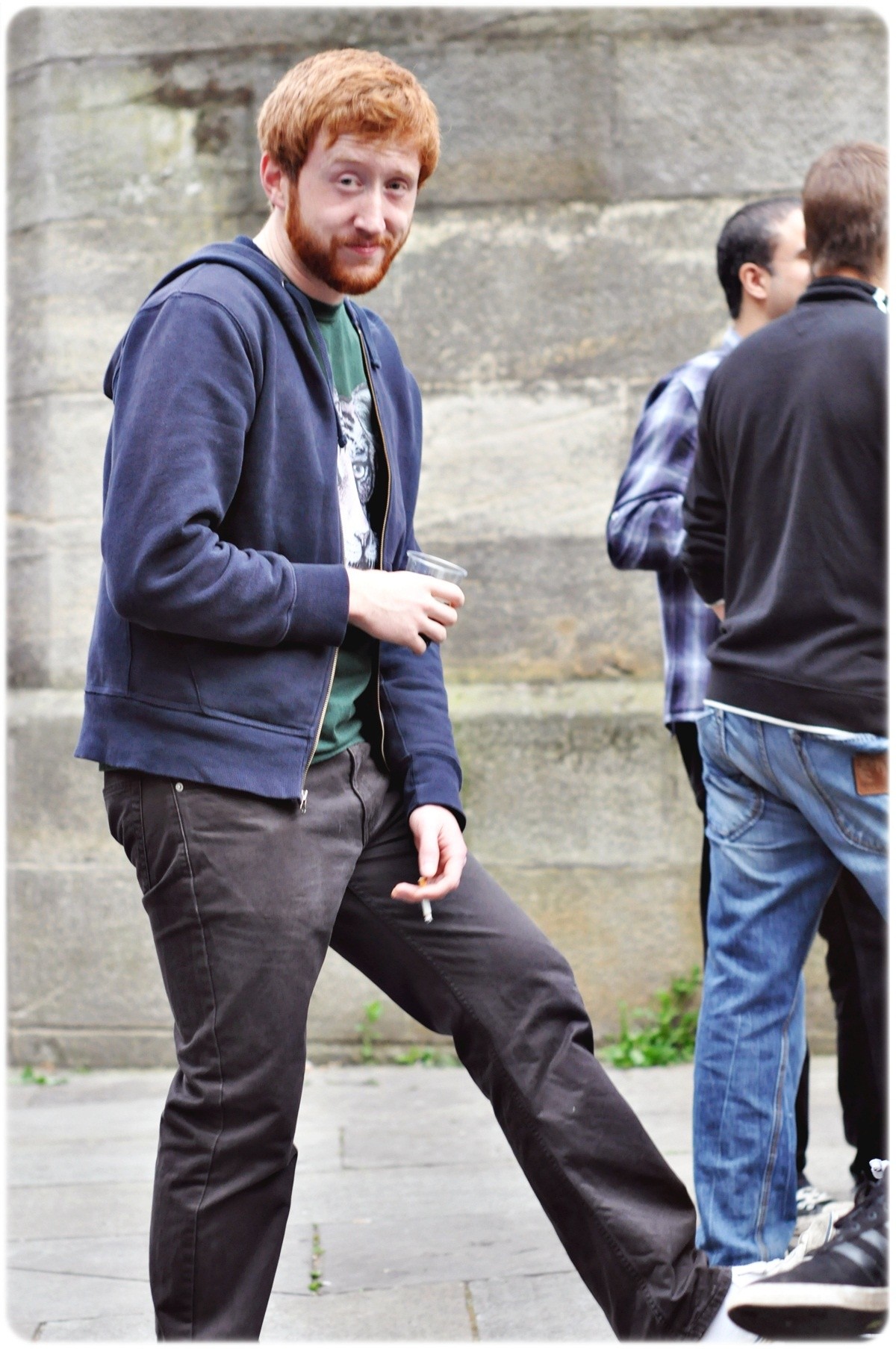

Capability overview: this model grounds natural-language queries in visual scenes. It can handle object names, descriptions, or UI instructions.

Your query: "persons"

[606,198,889,1210]
[732,1164,887,1336]
[681,139,888,1259]
[77,46,838,1344]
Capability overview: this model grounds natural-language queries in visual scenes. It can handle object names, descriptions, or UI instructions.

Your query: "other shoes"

[795,1181,844,1235]
[705,1212,836,1345]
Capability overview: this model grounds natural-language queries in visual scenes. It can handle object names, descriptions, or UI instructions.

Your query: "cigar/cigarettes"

[417,876,432,924]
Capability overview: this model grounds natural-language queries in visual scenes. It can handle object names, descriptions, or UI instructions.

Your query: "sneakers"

[725,1162,888,1339]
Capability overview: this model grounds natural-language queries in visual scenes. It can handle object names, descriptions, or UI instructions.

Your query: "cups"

[400,549,467,649]
